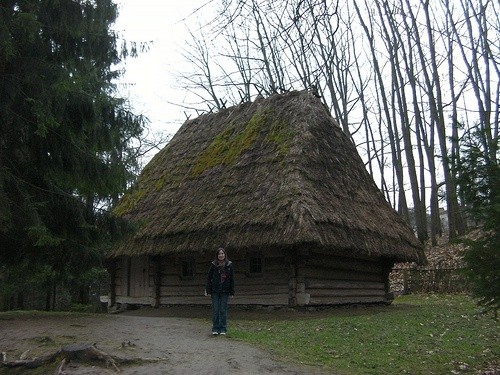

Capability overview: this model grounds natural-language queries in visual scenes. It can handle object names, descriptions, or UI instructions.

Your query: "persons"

[202,247,237,337]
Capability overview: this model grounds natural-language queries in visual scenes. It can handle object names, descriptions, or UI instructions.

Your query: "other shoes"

[212,332,225,335]
